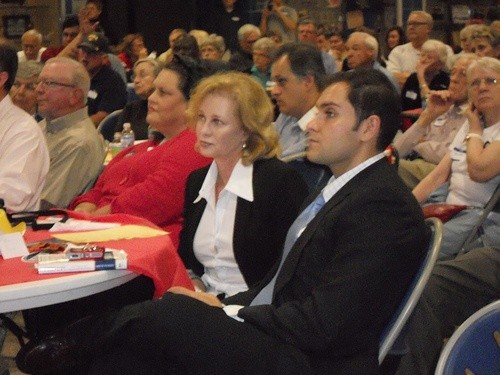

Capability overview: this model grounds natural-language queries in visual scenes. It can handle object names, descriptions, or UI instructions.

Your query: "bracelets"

[463,133,482,141]
[419,83,428,90]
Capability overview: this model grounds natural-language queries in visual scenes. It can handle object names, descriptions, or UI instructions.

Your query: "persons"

[27,68,215,328]
[0,0,397,214]
[72,71,307,320]
[17,67,432,375]
[388,0,500,375]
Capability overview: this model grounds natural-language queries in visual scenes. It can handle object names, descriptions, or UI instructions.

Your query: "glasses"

[33,79,75,90]
[469,79,497,88]
[407,22,427,26]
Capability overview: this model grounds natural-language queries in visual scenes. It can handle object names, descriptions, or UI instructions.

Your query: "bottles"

[111,132,124,158]
[121,122,135,149]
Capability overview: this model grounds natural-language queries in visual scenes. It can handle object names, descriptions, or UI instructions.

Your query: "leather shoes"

[15,310,113,375]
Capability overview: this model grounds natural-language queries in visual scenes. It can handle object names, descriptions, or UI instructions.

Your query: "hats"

[78,32,110,52]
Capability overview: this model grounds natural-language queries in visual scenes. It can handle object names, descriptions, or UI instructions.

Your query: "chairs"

[434,301,500,375]
[378,217,444,364]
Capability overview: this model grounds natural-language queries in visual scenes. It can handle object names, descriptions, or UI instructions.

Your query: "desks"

[0,207,195,348]
